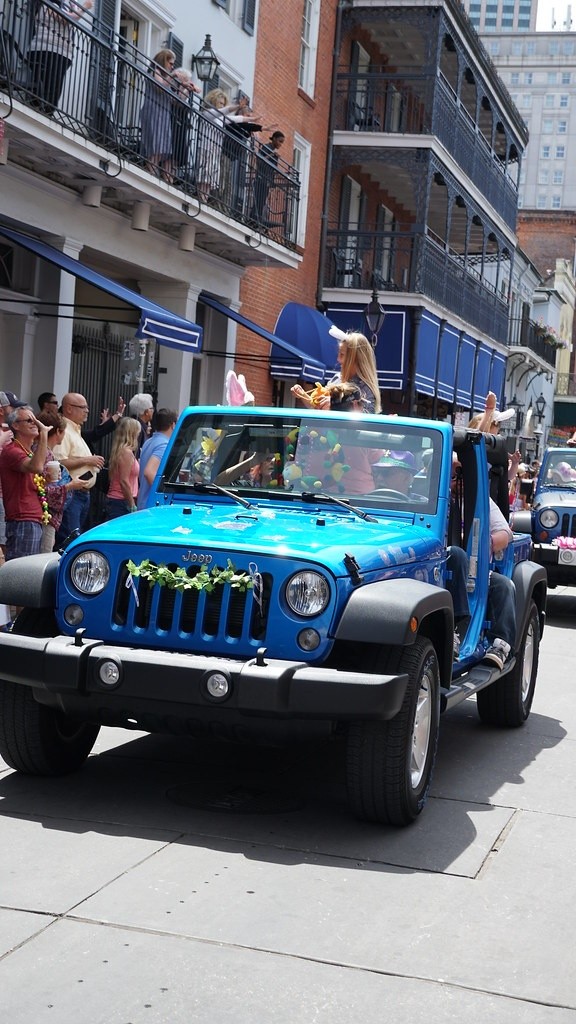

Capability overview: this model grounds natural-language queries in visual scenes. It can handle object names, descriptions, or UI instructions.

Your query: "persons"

[314,382,376,497]
[547,462,576,483]
[292,327,381,414]
[213,447,275,489]
[467,391,520,481]
[0,392,177,625]
[451,452,517,670]
[140,50,201,182]
[371,450,471,661]
[196,90,284,225]
[28,0,93,115]
[186,371,254,482]
[509,461,542,511]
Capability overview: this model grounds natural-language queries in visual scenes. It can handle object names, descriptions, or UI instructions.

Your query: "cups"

[93,466,100,473]
[46,461,61,482]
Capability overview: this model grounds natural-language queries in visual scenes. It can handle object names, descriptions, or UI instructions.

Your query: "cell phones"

[79,470,93,481]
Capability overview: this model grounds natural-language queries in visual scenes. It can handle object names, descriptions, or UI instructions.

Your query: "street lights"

[533,392,548,459]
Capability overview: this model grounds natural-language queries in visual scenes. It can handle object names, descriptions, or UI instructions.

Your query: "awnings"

[269,303,344,381]
[0,229,203,353]
[199,296,326,383]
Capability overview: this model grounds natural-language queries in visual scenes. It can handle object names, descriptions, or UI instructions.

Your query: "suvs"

[1,403,548,831]
[531,446,576,589]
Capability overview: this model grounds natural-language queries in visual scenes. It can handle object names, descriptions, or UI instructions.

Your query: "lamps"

[191,34,220,81]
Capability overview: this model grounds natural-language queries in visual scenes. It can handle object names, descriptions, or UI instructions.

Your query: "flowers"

[126,558,252,592]
[551,536,576,549]
[535,317,566,350]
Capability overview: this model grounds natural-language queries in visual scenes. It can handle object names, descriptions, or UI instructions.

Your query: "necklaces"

[15,439,52,525]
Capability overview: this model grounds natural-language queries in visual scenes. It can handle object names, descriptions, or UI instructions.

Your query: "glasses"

[450,475,456,481]
[492,421,500,426]
[149,407,155,412]
[70,404,89,409]
[15,418,33,423]
[46,401,58,405]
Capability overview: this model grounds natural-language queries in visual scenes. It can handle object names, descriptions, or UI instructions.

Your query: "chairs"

[355,103,381,131]
[97,108,220,204]
[411,476,427,496]
[243,178,286,229]
[0,29,47,110]
[332,248,399,292]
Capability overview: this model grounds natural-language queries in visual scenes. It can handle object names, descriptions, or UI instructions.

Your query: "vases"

[545,338,551,344]
[537,327,543,336]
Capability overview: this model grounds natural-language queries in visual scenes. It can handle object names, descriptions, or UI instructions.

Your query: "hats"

[0,391,28,407]
[479,408,515,422]
[567,431,576,444]
[330,385,372,404]
[370,449,419,476]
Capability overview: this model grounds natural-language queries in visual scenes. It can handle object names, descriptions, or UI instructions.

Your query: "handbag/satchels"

[94,453,111,494]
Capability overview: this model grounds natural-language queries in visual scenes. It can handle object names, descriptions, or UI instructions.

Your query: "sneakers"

[453,627,460,657]
[482,638,511,670]
